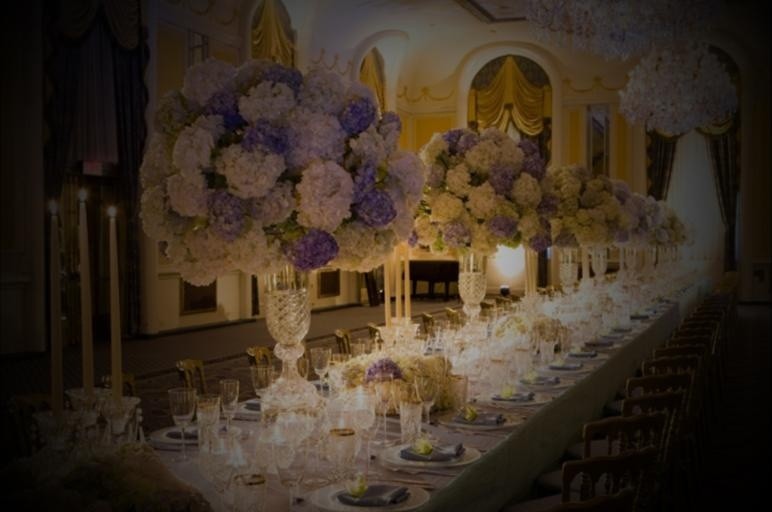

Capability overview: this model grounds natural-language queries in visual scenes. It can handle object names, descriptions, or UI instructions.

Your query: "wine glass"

[167,387,196,466]
[196,393,222,460]
[218,255,691,512]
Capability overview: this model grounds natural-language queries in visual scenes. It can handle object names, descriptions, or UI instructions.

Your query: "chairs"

[509,281,736,509]
[128,277,612,439]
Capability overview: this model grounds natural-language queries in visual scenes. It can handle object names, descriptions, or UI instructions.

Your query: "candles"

[107,207,123,417]
[47,200,63,417]
[76,189,94,408]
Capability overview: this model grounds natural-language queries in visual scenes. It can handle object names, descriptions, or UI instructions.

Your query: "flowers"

[550,164,684,251]
[405,124,552,256]
[617,59,737,138]
[138,59,426,274]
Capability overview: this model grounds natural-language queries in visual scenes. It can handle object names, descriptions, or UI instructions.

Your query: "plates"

[150,423,243,445]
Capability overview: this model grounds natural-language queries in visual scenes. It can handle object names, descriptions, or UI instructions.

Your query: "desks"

[121,273,715,512]
